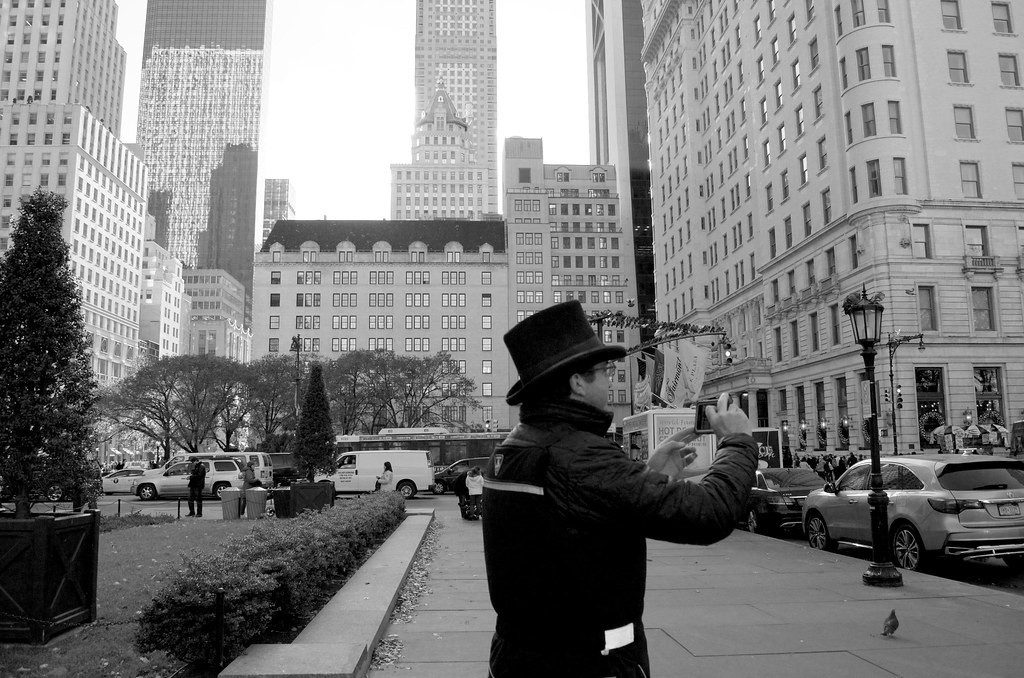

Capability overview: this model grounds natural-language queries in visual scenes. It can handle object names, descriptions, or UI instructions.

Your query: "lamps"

[885,408,893,428]
[783,414,853,434]
[962,406,972,426]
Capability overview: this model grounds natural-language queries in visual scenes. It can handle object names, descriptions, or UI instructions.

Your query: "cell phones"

[694,400,729,433]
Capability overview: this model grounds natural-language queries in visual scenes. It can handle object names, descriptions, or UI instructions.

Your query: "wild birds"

[882,609,900,637]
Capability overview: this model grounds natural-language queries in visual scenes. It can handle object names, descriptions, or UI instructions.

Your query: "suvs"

[801,453,1024,573]
[269,453,302,487]
[0,454,103,502]
[428,457,489,495]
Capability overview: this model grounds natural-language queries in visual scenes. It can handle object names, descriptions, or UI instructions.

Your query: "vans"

[141,452,274,496]
[313,450,435,500]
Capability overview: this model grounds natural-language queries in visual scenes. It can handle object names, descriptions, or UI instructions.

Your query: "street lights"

[843,281,904,588]
[289,333,303,437]
[885,330,925,454]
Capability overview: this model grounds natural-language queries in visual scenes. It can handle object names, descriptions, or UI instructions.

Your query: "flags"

[636,340,708,409]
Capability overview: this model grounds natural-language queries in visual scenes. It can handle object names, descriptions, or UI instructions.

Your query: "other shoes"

[476,516,479,520]
[196,514,202,517]
[185,511,195,517]
[469,515,473,521]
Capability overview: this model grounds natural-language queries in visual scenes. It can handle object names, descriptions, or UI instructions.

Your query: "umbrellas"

[931,423,1008,448]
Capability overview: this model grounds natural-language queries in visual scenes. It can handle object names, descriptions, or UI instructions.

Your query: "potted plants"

[289,363,338,518]
[0,184,102,647]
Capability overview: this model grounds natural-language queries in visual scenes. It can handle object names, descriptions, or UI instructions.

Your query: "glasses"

[592,360,616,379]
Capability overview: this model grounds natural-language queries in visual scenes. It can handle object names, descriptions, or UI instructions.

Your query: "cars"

[130,459,245,501]
[102,468,146,495]
[742,467,827,538]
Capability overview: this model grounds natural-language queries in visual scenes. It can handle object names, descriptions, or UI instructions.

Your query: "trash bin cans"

[273,490,290,518]
[220,487,241,519]
[245,487,268,519]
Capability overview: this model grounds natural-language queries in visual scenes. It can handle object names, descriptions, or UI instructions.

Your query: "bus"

[620,408,717,485]
[330,427,511,473]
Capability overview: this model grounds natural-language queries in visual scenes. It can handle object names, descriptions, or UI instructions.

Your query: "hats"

[246,461,255,467]
[502,300,627,406]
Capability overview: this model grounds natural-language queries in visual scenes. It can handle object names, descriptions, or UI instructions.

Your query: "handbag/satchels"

[829,462,833,470]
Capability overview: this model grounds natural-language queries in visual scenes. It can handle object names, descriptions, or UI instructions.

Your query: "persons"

[480,301,758,678]
[240,461,258,515]
[450,466,484,520]
[375,461,393,492]
[794,452,867,483]
[116,459,127,470]
[184,456,206,517]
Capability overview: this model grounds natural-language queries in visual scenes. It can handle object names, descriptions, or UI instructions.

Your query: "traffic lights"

[896,393,904,409]
[884,388,890,402]
